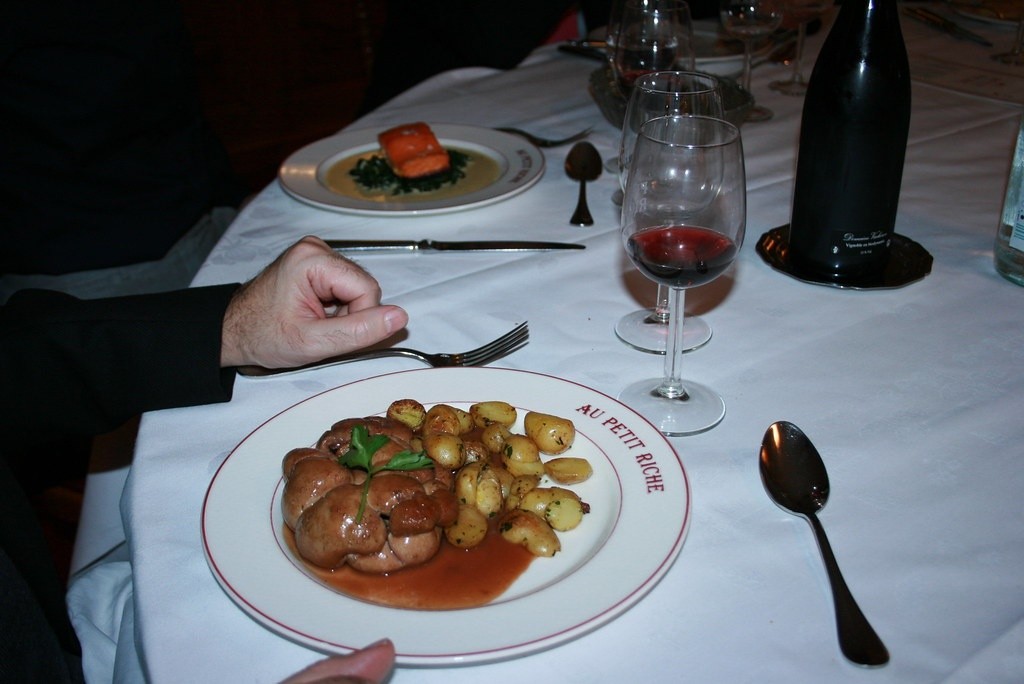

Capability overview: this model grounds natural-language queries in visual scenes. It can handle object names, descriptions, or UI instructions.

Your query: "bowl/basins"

[676,32,748,78]
[590,68,757,136]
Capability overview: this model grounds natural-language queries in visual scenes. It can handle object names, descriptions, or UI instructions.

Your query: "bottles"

[786,0,911,271]
[993,112,1024,288]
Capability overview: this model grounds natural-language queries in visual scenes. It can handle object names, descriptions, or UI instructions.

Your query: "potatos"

[386,399,592,556]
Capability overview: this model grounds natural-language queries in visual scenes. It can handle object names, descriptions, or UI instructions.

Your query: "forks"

[235,321,529,367]
[491,126,591,148]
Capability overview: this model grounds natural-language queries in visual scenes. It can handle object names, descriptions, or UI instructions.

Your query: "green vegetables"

[338,423,435,525]
[348,150,472,196]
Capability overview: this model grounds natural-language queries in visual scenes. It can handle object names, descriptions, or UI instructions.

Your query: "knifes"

[901,6,993,47]
[325,239,586,254]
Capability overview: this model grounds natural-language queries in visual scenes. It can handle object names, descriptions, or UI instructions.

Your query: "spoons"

[758,422,891,667]
[564,142,602,227]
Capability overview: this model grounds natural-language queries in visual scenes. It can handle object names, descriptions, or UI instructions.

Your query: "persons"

[0,233,408,684]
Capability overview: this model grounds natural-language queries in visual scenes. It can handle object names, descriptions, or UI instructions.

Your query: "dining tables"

[68,0,1023,684]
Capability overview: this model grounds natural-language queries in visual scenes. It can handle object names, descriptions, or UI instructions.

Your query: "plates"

[278,123,546,216]
[201,365,693,667]
[951,0,1024,26]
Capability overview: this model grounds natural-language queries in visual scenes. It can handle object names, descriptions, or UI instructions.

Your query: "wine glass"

[621,115,747,439]
[601,1,833,172]
[616,69,729,353]
